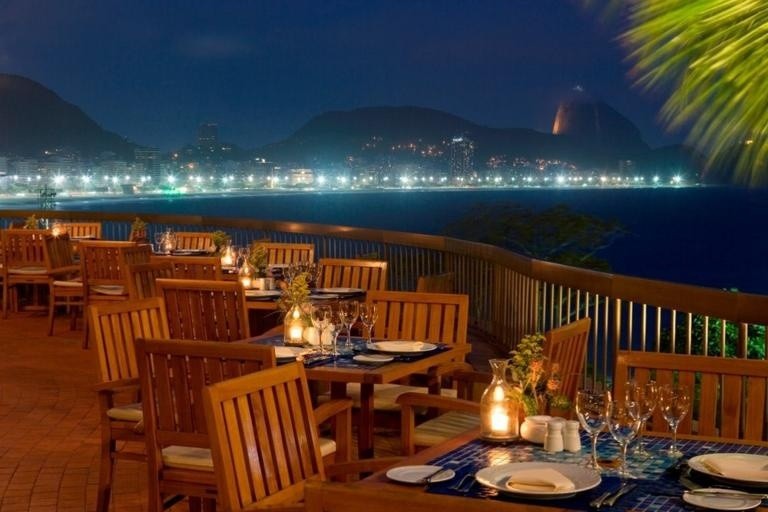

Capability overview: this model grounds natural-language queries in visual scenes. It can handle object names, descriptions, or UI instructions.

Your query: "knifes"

[589,481,637,509]
[684,490,768,501]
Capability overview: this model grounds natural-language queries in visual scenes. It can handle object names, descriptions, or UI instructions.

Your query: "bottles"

[519,415,582,453]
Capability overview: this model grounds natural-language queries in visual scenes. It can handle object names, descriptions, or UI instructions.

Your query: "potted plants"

[508,329,549,421]
[549,394,572,418]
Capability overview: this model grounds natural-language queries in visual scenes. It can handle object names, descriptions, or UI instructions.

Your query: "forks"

[678,478,700,490]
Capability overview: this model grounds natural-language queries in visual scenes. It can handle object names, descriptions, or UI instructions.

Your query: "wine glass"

[575,381,692,478]
[309,300,380,362]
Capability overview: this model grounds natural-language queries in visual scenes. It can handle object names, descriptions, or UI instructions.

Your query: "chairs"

[138,337,356,512]
[304,478,549,510]
[415,271,455,294]
[201,358,404,509]
[84,297,194,512]
[395,316,590,458]
[452,315,590,414]
[613,349,768,442]
[316,290,474,434]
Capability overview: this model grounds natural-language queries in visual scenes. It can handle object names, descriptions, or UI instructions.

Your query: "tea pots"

[479,358,523,444]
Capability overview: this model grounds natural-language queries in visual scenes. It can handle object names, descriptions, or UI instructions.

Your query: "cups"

[260,277,276,290]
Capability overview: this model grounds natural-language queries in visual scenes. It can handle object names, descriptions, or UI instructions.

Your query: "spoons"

[416,466,449,489]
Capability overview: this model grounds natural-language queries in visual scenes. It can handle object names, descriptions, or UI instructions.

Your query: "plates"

[366,340,438,355]
[687,452,768,483]
[383,464,456,484]
[682,488,762,511]
[474,462,603,501]
[352,354,394,364]
